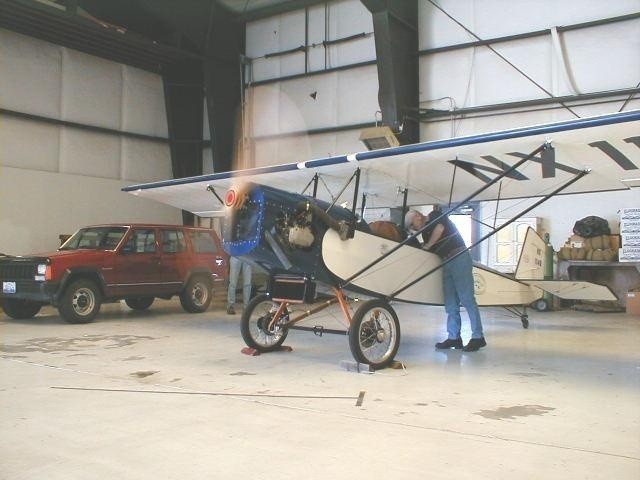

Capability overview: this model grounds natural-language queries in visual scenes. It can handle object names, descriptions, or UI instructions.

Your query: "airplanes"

[121,111,640,366]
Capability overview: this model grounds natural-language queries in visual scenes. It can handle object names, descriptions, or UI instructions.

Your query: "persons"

[403,209,487,353]
[225,253,252,315]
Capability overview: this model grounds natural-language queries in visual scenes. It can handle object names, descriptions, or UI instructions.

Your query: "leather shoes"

[227,309,235,314]
[463,337,486,352]
[435,338,463,349]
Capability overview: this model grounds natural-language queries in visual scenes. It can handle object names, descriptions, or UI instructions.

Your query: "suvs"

[0,223,230,323]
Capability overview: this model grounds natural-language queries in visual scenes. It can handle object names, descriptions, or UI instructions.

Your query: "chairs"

[122,231,164,252]
